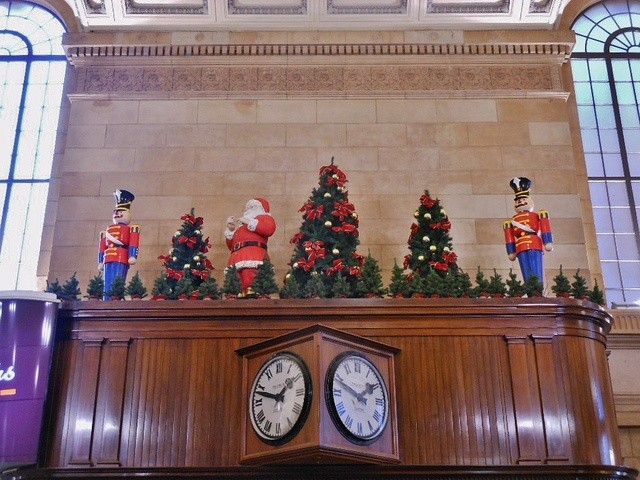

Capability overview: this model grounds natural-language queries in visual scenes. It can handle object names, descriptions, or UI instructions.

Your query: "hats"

[113,190,134,211]
[246,198,269,213]
[509,176,532,200]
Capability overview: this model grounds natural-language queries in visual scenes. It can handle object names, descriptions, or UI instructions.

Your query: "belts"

[108,244,128,248]
[516,232,537,238]
[230,241,268,254]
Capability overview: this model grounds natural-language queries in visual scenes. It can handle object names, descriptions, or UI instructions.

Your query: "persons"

[224,197,276,299]
[98,188,141,302]
[503,176,553,298]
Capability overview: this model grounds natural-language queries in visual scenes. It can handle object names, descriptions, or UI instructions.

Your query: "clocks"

[322,349,390,447]
[247,349,314,448]
